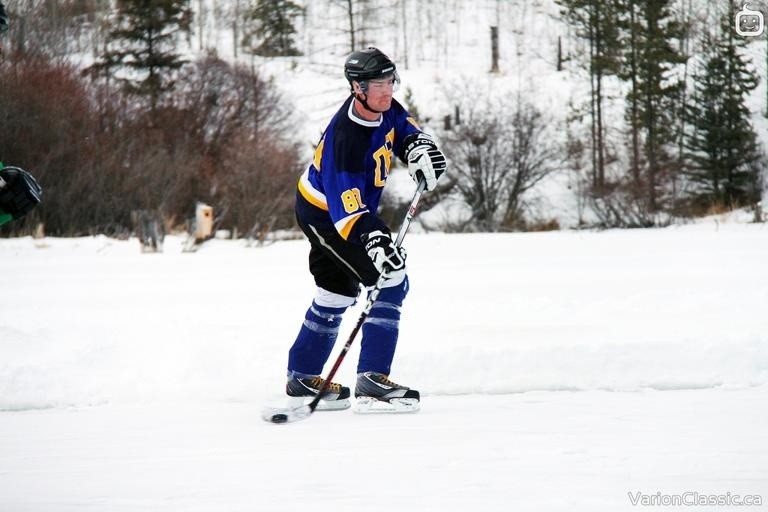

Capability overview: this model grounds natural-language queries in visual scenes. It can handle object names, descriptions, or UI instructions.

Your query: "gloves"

[397,132,448,194]
[358,229,407,279]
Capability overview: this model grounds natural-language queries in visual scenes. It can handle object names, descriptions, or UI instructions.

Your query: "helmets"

[344,47,396,82]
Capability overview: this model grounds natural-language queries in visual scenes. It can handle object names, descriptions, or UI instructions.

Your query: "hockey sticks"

[262,178,426,422]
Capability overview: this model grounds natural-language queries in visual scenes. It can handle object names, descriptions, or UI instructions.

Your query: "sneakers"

[286,369,350,402]
[355,371,419,400]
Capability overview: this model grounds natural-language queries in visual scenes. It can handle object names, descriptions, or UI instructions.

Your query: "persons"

[285,46,447,406]
[0,159,42,226]
